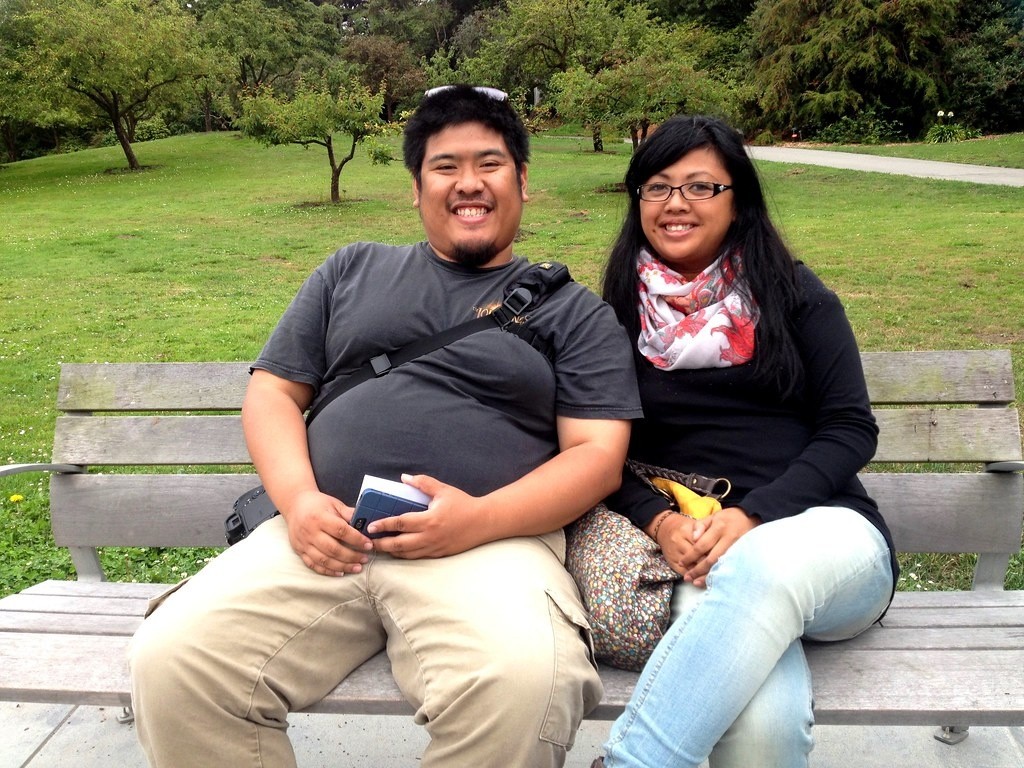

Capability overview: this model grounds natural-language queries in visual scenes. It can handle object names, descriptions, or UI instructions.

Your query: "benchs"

[0,349,1024,768]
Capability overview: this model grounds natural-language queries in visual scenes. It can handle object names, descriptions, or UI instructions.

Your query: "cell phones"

[348,489,428,539]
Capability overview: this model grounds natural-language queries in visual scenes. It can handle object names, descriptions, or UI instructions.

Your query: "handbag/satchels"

[224,484,281,547]
[566,457,732,674]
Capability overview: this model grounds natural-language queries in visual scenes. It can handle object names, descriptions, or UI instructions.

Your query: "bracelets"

[651,510,677,542]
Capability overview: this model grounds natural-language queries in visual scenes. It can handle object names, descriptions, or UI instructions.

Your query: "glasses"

[635,182,733,201]
[420,84,509,105]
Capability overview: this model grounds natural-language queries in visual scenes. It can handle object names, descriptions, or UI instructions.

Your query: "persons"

[591,114,901,765]
[122,84,650,768]
